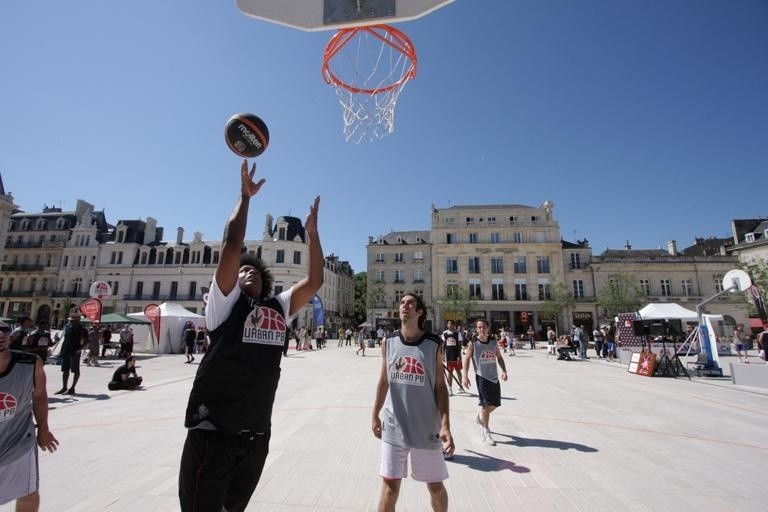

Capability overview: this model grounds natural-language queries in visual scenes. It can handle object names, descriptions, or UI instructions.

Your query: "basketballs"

[224,113,269,158]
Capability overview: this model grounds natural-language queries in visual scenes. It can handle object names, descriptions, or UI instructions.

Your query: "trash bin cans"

[744,336,752,350]
[367,339,375,348]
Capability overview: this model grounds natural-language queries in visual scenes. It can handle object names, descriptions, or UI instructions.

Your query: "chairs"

[570,344,577,360]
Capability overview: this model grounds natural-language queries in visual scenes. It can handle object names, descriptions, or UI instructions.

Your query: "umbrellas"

[68,312,151,325]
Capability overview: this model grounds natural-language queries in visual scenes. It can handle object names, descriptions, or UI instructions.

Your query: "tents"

[126,302,206,354]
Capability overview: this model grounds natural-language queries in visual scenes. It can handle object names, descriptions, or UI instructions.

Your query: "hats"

[66,313,81,321]
[600,324,607,329]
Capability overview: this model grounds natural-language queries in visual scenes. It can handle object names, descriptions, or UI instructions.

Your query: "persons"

[371,292,455,512]
[457,324,478,354]
[179,160,324,512]
[557,323,616,362]
[462,318,507,446]
[499,327,516,356]
[196,328,205,354]
[283,324,395,356]
[758,323,768,361]
[9,312,142,396]
[441,320,463,395]
[526,325,536,349]
[184,324,196,363]
[546,326,556,354]
[733,323,749,362]
[0,317,59,512]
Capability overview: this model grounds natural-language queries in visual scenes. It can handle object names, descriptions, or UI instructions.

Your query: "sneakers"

[448,387,453,395]
[55,387,75,396]
[738,357,749,363]
[457,384,463,394]
[480,429,497,446]
[185,357,195,363]
[596,354,617,362]
[474,413,490,432]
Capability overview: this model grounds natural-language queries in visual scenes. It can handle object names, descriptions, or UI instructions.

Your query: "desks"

[101,341,140,358]
[546,344,571,359]
